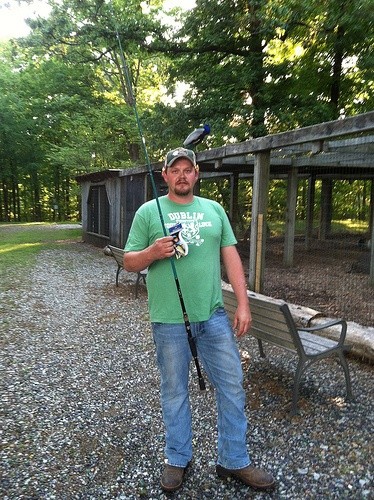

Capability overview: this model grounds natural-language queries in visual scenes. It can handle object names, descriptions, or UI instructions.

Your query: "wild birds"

[182,122,211,152]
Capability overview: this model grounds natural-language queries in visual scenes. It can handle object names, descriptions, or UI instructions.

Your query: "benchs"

[218,284,355,419]
[107,245,148,302]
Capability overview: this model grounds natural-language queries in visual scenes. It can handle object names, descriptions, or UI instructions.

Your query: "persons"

[124,147,274,491]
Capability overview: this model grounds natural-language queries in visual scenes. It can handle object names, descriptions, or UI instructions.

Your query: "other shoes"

[161,456,192,490]
[216,464,274,488]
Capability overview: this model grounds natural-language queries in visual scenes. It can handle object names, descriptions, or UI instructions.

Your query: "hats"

[165,147,197,170]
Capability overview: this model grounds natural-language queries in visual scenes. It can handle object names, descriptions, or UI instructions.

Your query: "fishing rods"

[115,33,207,392]
